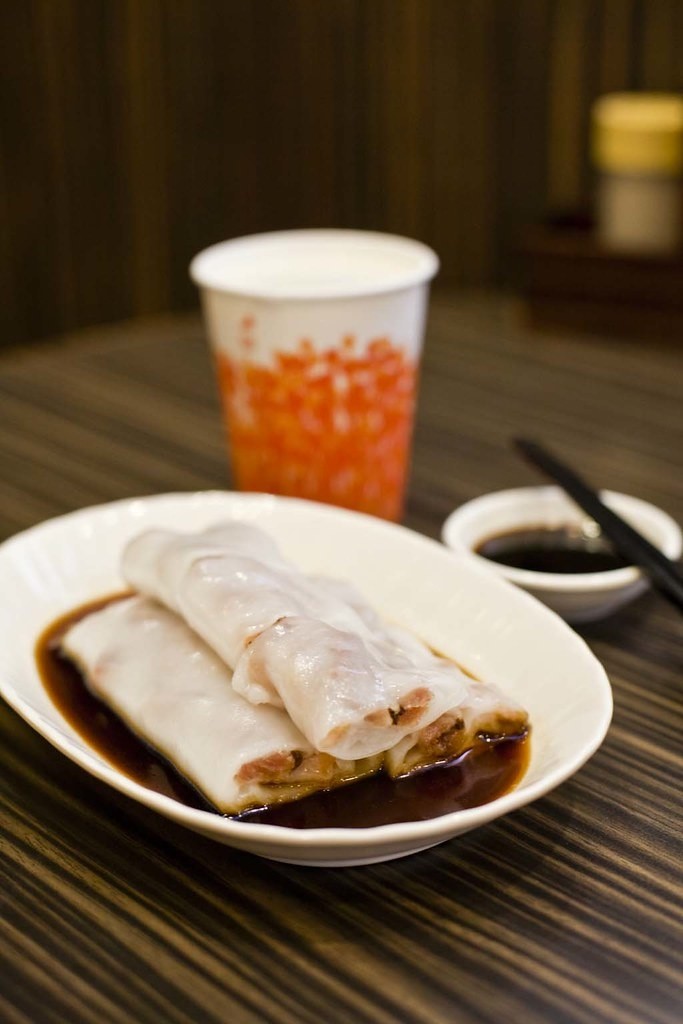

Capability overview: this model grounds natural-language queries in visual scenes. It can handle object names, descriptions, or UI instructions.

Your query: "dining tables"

[0,327,683,1024]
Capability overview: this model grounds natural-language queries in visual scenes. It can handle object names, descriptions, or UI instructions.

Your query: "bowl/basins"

[440,484,683,624]
[0,488,615,869]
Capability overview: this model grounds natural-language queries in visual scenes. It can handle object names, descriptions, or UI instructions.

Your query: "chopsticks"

[515,439,683,614]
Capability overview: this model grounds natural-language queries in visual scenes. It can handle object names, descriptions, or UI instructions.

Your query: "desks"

[494,220,683,350]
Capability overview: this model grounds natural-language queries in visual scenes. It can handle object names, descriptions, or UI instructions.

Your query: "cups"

[187,226,440,526]
